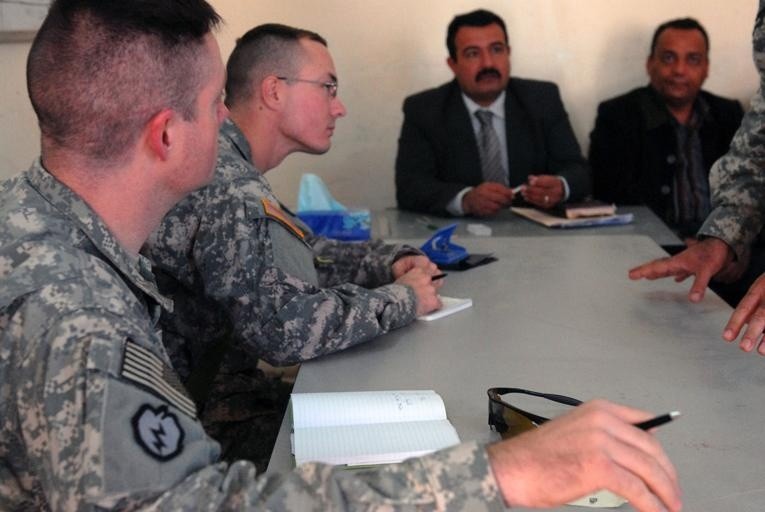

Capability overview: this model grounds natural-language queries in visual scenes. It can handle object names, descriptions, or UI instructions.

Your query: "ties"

[474,111,505,185]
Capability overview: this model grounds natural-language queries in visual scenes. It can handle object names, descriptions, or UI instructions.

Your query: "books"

[418,295,472,321]
[508,206,634,229]
[290,389,461,468]
[550,195,616,217]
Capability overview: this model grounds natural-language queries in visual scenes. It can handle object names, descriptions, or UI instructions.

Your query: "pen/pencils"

[636,410,683,431]
[511,184,526,194]
[431,273,448,281]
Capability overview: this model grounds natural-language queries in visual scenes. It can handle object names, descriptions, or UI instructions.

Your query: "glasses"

[487,385,584,440]
[277,76,337,97]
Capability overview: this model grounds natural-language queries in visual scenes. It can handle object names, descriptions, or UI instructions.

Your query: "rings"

[545,196,549,203]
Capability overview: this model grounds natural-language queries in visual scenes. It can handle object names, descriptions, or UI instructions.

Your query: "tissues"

[295,172,372,241]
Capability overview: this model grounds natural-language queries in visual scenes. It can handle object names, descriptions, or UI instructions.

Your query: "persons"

[0,0,681,512]
[139,24,445,474]
[588,17,765,310]
[396,9,592,219]
[629,0,765,356]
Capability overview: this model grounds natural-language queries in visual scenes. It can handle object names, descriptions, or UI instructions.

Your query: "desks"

[354,205,685,248]
[267,235,764,512]
